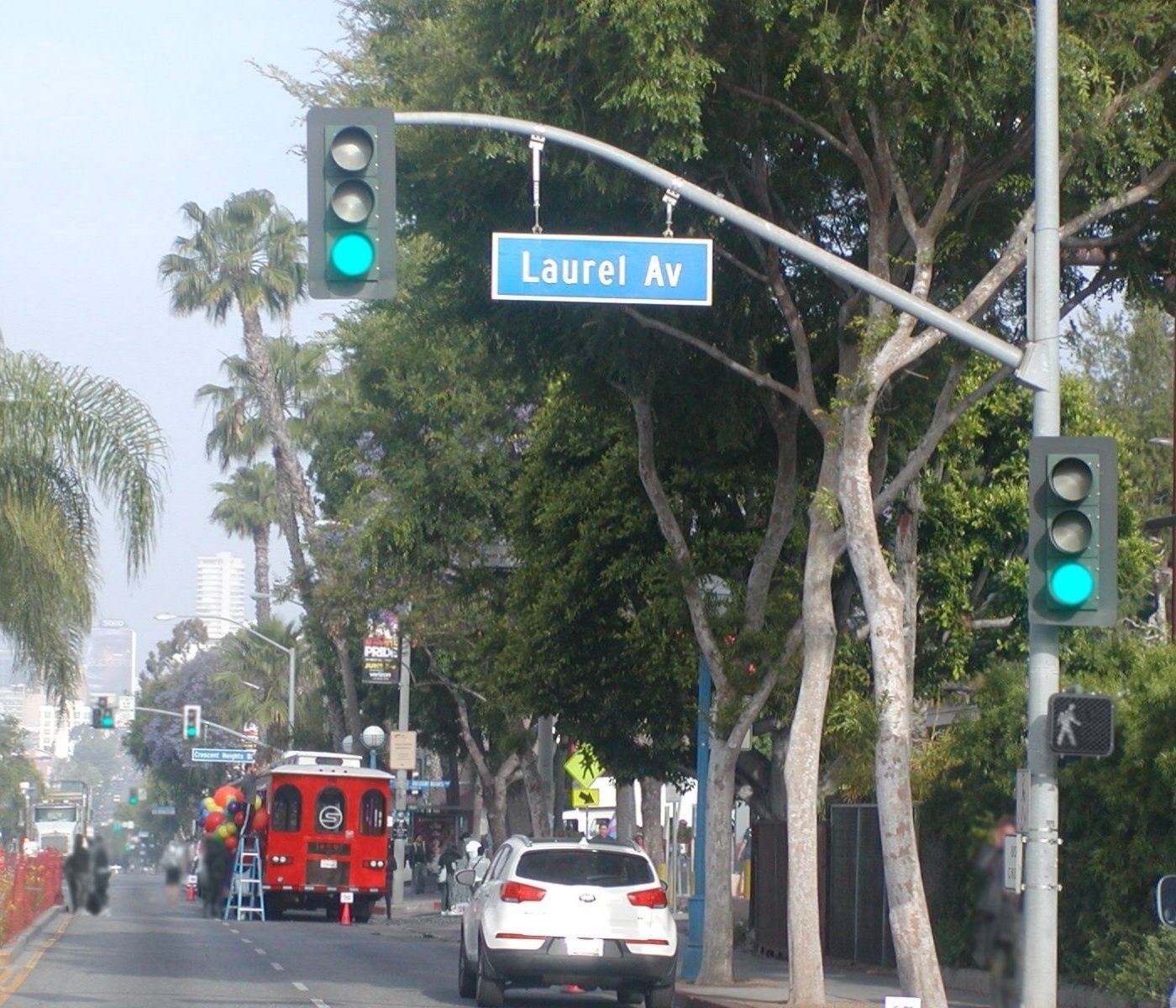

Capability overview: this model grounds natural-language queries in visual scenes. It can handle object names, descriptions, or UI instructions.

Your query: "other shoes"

[441,909,447,916]
[416,889,425,894]
[448,911,456,916]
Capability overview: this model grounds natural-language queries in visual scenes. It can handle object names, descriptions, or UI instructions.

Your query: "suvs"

[458,832,677,1007]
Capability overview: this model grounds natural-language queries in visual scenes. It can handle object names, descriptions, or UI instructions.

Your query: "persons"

[968,812,1076,1008]
[660,818,692,858]
[738,827,752,896]
[383,832,492,920]
[201,838,233,919]
[65,834,110,919]
[165,841,181,903]
[635,832,644,849]
[592,823,614,845]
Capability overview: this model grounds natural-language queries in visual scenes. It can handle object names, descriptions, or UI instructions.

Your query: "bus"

[223,750,396,923]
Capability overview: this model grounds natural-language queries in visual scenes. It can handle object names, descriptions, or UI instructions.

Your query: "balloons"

[198,787,268,867]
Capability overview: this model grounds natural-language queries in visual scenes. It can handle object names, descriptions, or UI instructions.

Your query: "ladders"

[225,834,264,921]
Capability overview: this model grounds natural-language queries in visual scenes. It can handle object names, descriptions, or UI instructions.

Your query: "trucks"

[12,779,96,861]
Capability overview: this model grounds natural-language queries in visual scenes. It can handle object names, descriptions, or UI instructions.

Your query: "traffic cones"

[186,885,196,902]
[340,903,351,926]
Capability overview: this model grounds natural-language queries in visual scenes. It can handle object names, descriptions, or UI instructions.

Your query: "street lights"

[359,725,390,769]
[153,613,297,750]
[314,519,412,919]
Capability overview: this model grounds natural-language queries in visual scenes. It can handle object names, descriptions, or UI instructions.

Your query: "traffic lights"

[1028,435,1118,627]
[183,704,201,740]
[92,697,113,729]
[307,106,397,299]
[129,787,138,805]
[1046,694,1115,757]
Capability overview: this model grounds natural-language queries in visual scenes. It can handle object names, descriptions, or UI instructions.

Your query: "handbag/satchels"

[435,865,447,884]
